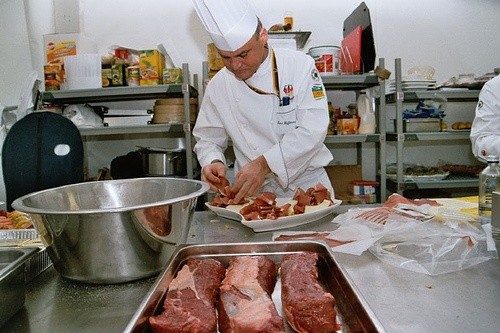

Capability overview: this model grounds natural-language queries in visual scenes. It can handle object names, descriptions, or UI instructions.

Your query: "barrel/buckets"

[309,45,343,76]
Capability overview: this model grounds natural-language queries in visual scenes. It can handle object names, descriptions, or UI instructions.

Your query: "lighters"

[283,97,290,106]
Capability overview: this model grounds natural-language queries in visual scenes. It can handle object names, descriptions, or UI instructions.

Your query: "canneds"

[127,66,139,87]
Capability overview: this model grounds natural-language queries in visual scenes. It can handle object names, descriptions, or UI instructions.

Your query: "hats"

[193,0,259,52]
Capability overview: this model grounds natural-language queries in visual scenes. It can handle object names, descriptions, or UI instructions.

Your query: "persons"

[192,0,336,205]
[470,72,500,164]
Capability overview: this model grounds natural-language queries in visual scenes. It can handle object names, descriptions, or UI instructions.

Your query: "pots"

[147,148,187,178]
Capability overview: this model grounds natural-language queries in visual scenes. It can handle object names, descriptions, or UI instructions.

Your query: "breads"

[451,122,471,128]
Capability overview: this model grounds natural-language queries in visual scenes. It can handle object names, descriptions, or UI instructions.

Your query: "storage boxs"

[158,52,166,85]
[42,31,99,89]
[138,49,160,85]
[390,119,442,133]
[347,184,373,196]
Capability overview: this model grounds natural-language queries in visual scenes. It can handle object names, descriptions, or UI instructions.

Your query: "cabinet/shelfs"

[375,57,482,196]
[202,57,387,204]
[30,62,200,180]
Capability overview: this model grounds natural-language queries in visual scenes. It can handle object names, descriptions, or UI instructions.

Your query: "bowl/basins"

[336,118,358,135]
[11,177,211,285]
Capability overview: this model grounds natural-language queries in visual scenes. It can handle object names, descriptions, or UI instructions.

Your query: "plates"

[203,198,342,232]
[377,169,449,182]
[442,164,487,176]
[389,77,437,92]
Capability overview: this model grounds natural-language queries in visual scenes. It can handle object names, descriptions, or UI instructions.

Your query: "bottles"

[356,91,376,134]
[479,155,500,224]
[326,101,335,134]
